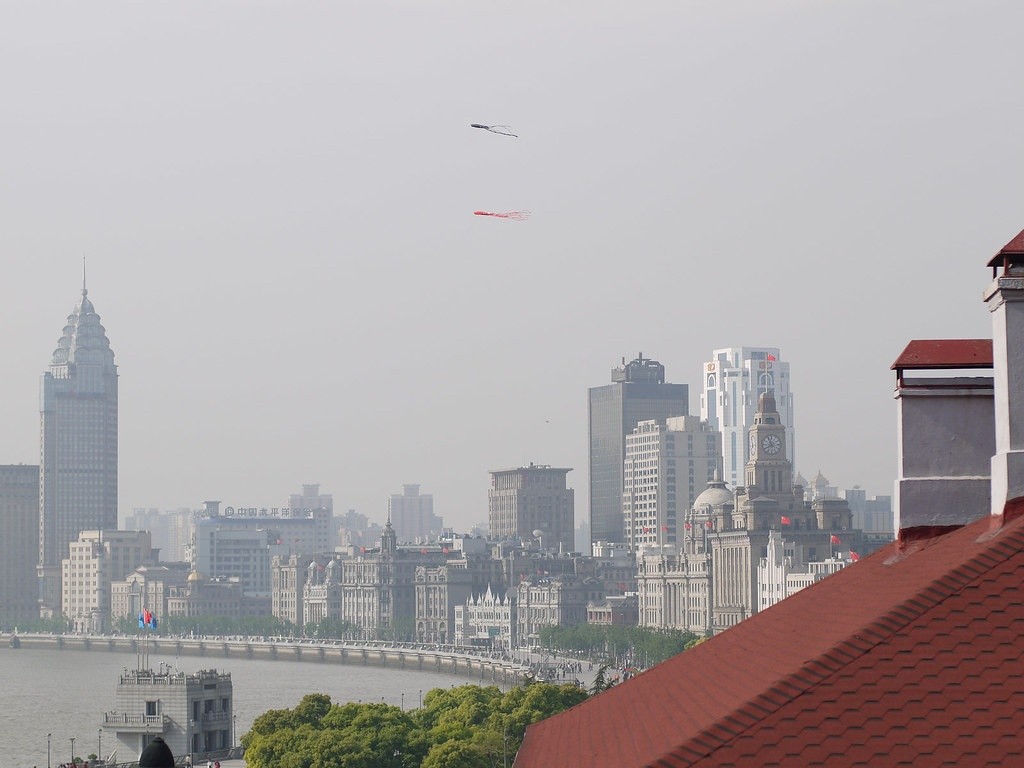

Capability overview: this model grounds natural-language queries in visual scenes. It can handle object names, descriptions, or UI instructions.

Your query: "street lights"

[47,732,51,768]
[419,690,422,711]
[402,693,405,711]
[97,729,103,765]
[145,722,151,747]
[233,714,238,747]
[190,717,195,768]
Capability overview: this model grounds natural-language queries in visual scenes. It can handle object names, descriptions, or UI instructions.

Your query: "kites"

[471,123,518,137]
[473,210,532,220]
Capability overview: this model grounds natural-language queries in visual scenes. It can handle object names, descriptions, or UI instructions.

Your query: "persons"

[208,758,212,768]
[553,653,643,689]
[186,755,191,768]
[215,762,220,768]
[59,761,89,768]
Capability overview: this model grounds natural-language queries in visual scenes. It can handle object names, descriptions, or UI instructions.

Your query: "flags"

[662,527,669,532]
[421,549,427,554]
[318,560,327,571]
[644,528,648,532]
[831,535,841,544]
[138,609,157,629]
[685,523,693,529]
[782,516,790,525]
[706,521,713,527]
[443,547,449,553]
[768,355,775,362]
[851,551,860,559]
[277,539,284,545]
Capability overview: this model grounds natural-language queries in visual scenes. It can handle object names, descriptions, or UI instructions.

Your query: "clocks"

[750,435,756,457]
[762,434,782,455]
